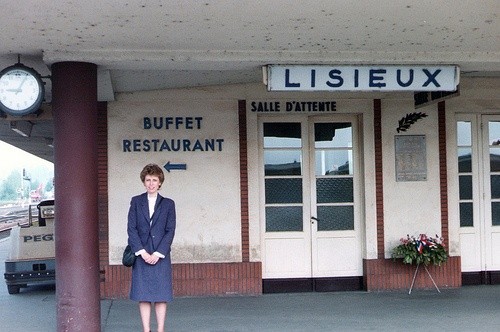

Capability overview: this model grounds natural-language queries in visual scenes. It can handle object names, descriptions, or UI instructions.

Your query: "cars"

[3,199,56,294]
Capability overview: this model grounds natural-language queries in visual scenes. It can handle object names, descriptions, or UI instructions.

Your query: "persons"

[128,163,176,332]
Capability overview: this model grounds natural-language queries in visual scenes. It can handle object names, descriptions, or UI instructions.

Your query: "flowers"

[392,234,449,266]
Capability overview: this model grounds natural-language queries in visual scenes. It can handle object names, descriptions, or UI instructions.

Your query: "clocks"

[0,63,46,117]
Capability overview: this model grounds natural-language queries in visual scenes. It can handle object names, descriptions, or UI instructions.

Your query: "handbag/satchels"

[122,244,136,266]
